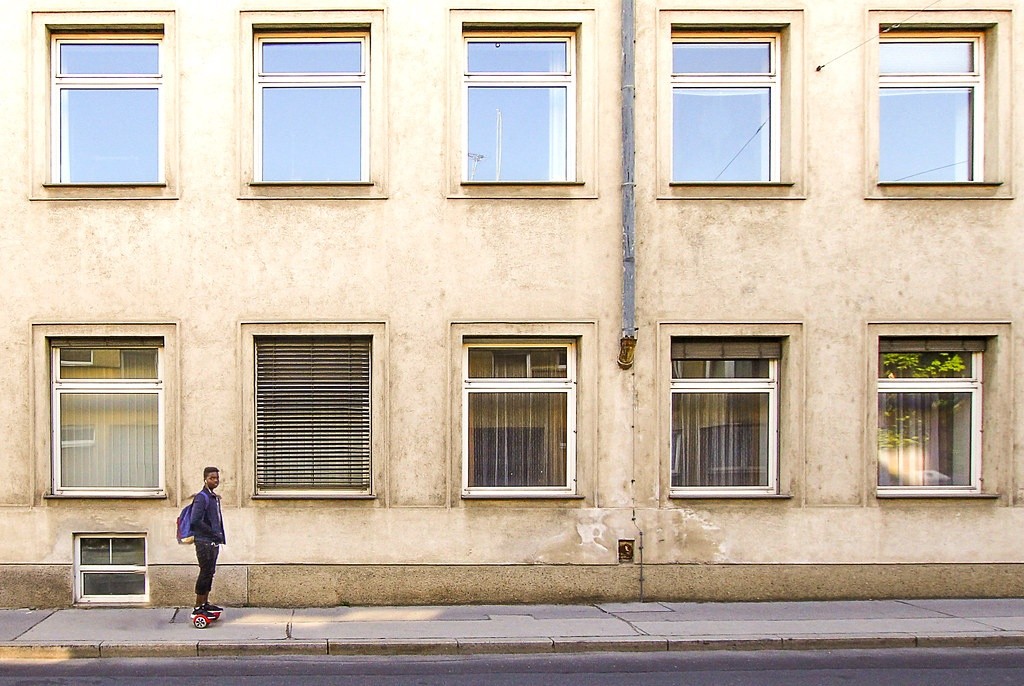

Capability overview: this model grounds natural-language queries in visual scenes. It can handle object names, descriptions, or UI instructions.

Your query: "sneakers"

[190,602,225,619]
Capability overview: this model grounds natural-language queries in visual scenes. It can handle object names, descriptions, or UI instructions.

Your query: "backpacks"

[176,492,210,545]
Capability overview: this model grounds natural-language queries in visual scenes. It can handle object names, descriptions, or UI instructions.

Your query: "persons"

[190,467,226,619]
